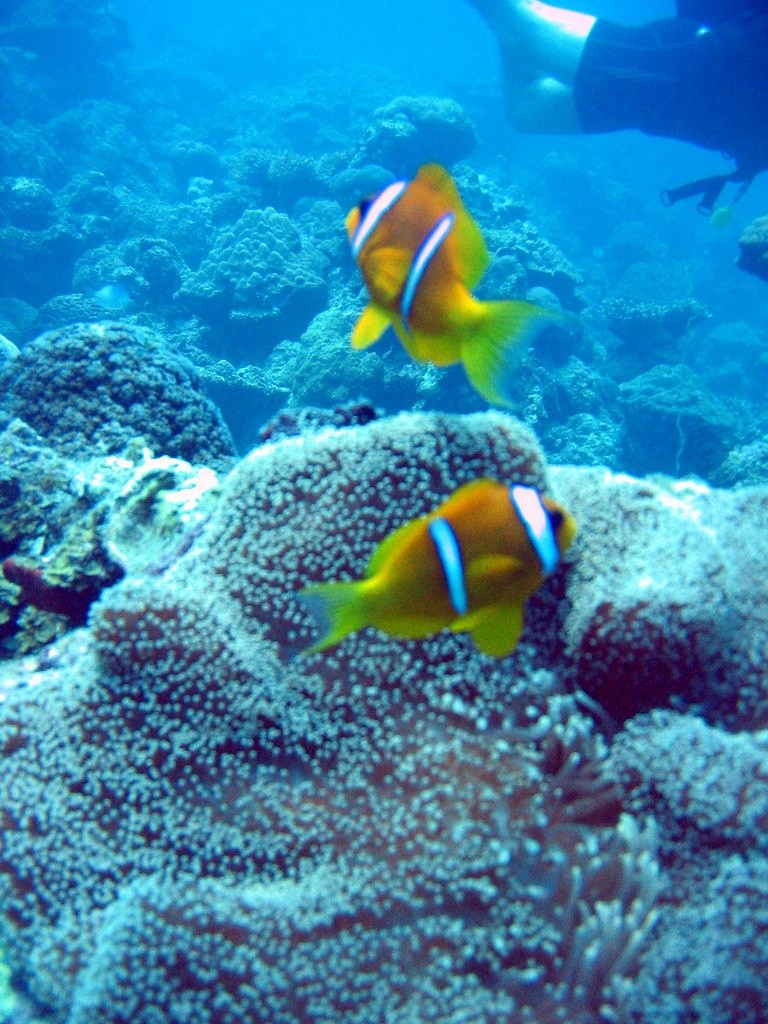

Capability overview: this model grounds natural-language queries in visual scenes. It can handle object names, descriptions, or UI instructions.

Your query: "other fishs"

[283,478,579,664]
[345,160,564,413]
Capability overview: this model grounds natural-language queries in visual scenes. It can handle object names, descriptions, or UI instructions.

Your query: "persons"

[464,0,768,172]
